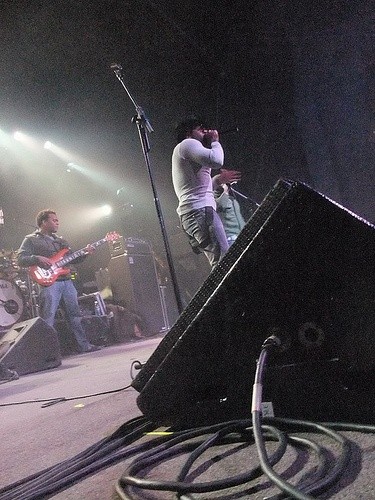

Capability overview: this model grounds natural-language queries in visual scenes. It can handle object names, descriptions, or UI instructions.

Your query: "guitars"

[30,231,122,287]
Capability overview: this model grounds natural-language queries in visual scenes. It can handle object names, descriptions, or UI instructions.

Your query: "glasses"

[196,124,203,131]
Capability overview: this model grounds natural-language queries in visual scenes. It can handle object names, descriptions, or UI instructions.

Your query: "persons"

[172,114,242,270]
[208,162,246,246]
[15,208,102,354]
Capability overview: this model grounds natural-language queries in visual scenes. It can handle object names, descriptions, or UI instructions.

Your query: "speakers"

[109,253,168,339]
[0,316,61,380]
[127,178,375,430]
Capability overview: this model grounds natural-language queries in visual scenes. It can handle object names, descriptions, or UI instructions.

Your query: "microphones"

[7,303,16,310]
[218,128,239,134]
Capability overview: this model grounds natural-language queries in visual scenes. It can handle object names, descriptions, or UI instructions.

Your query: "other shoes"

[79,344,102,353]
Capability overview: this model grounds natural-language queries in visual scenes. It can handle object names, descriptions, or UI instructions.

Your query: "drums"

[0,276,27,329]
[0,249,21,280]
